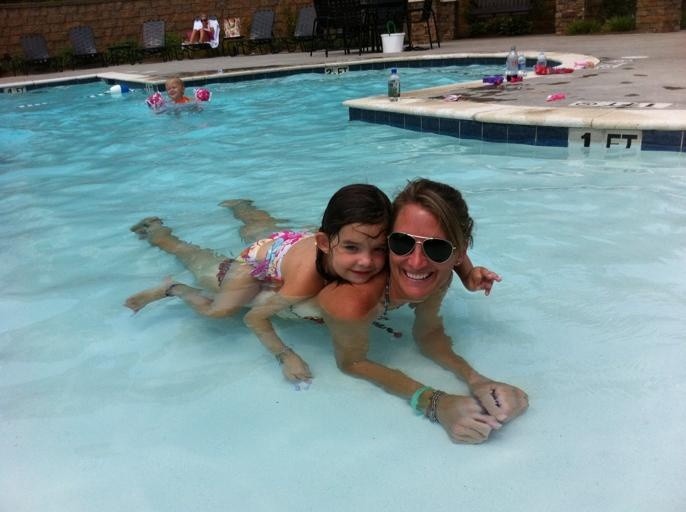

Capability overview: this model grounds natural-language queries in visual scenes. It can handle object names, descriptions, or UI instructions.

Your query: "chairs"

[241,8,278,55]
[22,35,64,74]
[281,8,320,53]
[68,25,107,71]
[131,21,168,64]
[310,1,440,58]
[188,15,220,59]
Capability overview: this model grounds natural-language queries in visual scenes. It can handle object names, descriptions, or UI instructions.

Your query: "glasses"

[200,19,207,22]
[384,230,455,264]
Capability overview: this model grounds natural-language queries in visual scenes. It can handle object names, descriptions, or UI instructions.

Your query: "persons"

[128,177,531,446]
[185,13,213,45]
[122,185,394,388]
[158,76,200,107]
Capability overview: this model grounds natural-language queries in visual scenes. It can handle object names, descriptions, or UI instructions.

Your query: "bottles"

[506,44,519,82]
[386,68,402,103]
[517,52,528,80]
[536,52,547,67]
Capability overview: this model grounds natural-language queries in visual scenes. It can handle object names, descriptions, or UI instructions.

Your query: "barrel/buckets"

[381,24,404,56]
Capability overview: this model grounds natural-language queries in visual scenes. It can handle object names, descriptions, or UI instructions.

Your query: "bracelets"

[424,389,447,425]
[408,386,433,416]
[275,346,293,365]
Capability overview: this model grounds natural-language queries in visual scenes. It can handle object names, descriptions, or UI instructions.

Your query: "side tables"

[168,43,194,60]
[108,46,132,66]
[221,36,246,57]
